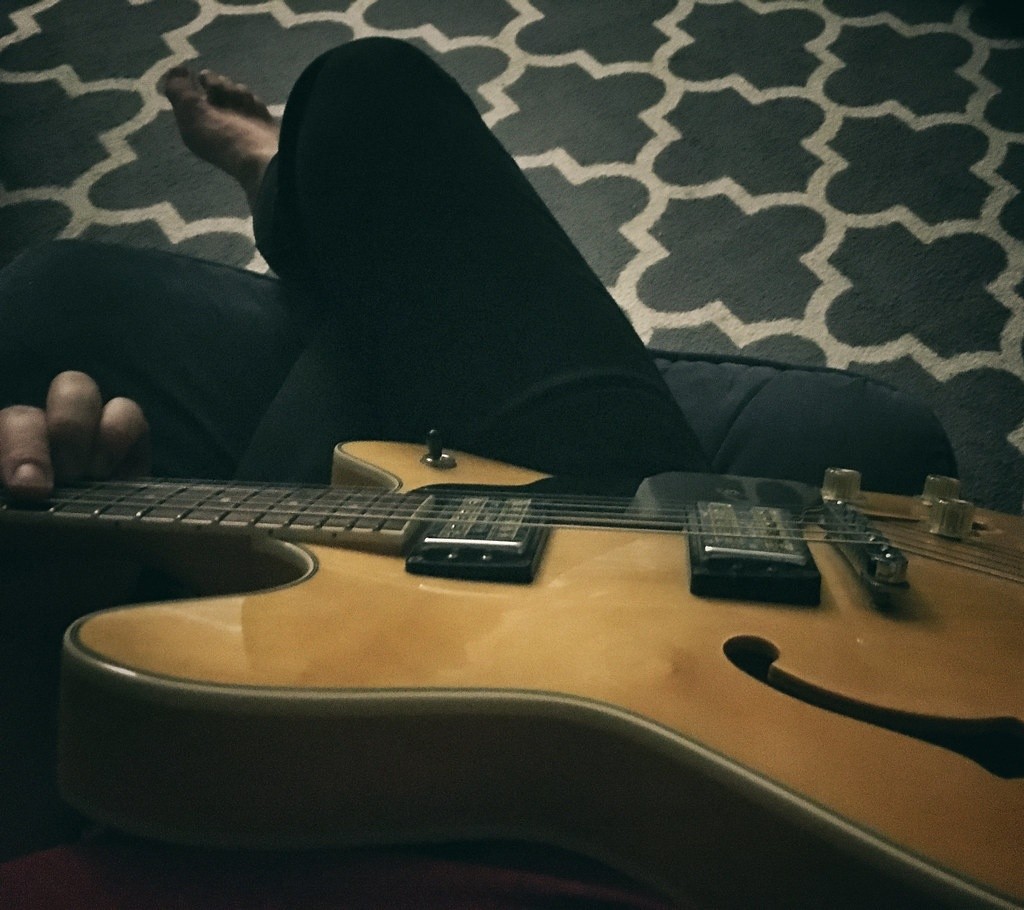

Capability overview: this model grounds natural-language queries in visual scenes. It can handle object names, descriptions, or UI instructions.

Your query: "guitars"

[2,439,1022,910]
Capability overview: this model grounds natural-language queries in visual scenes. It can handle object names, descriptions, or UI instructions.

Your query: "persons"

[1,36,948,910]
